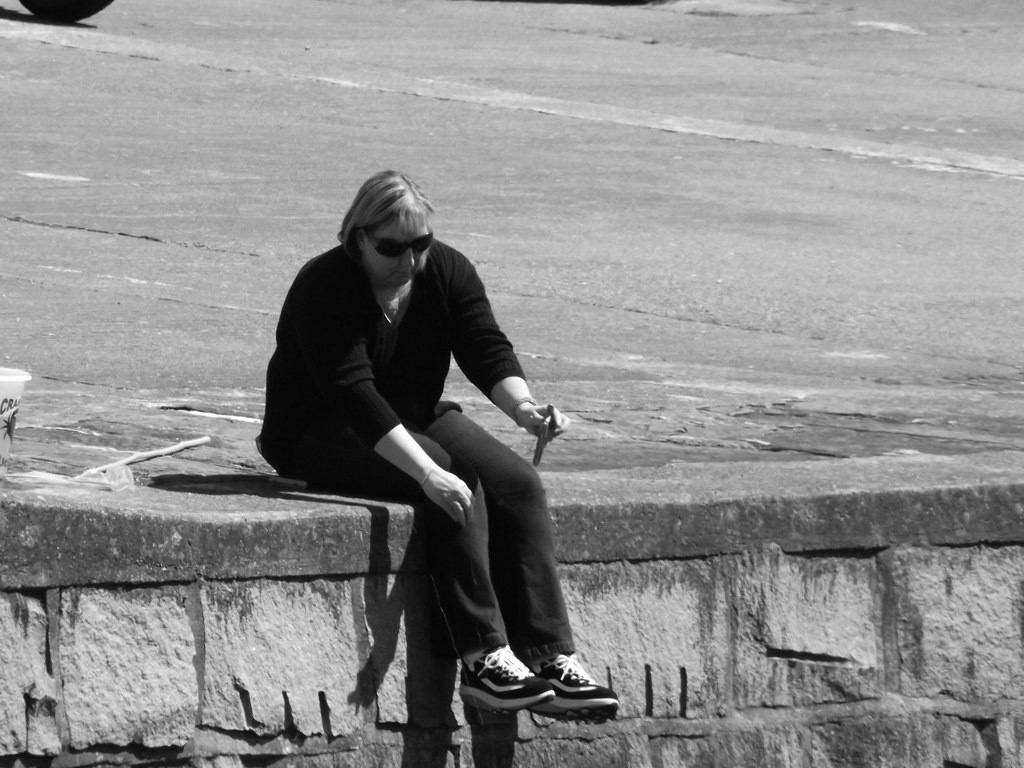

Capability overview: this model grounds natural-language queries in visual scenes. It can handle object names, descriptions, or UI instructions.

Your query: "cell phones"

[533,404,556,466]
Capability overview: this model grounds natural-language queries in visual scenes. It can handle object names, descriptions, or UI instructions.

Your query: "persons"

[254,170,619,724]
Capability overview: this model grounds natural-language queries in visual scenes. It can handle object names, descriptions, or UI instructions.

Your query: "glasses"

[355,225,433,257]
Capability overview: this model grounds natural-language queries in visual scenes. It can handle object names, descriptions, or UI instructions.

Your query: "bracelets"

[420,465,438,486]
[512,396,536,426]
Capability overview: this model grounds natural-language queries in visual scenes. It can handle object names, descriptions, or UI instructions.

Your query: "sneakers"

[525,653,619,725]
[459,644,555,715]
